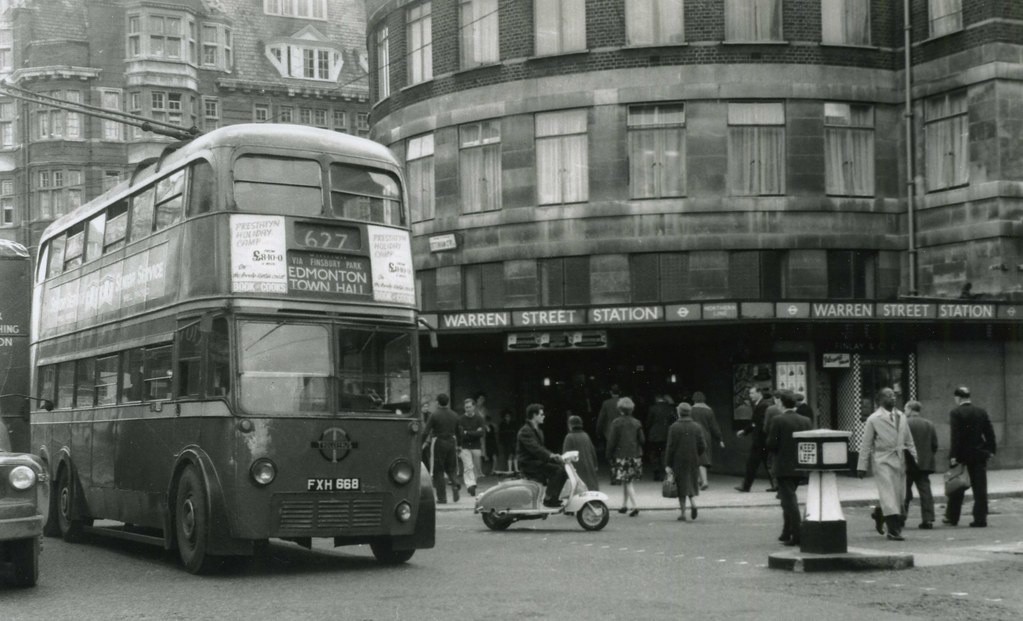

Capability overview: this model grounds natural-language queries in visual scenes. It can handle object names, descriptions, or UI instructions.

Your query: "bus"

[30,120,435,580]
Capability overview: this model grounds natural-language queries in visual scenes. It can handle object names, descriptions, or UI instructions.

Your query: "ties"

[889,413,894,422]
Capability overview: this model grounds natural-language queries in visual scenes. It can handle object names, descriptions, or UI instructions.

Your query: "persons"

[943,386,997,528]
[481,407,519,473]
[516,404,569,508]
[901,402,938,529]
[396,390,492,504]
[598,384,726,520]
[764,389,814,547]
[857,388,917,540]
[562,416,599,491]
[734,382,816,492]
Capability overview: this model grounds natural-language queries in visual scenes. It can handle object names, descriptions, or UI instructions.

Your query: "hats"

[677,402,692,416]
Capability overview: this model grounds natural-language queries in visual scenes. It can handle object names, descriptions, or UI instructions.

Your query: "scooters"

[473,450,610,531]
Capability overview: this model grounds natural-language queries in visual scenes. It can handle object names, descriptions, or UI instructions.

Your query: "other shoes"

[618,507,627,514]
[733,487,749,492]
[654,475,663,481]
[699,480,709,490]
[778,534,790,540]
[544,499,563,507]
[436,500,447,503]
[887,533,905,540]
[468,486,475,496]
[766,488,776,492]
[969,520,987,527]
[678,516,685,521]
[783,537,799,546]
[942,519,957,525]
[629,508,639,517]
[919,521,932,529]
[691,508,697,520]
[453,486,459,502]
[871,513,885,535]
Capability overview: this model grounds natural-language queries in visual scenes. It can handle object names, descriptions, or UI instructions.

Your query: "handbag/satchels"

[943,463,972,497]
[662,472,678,498]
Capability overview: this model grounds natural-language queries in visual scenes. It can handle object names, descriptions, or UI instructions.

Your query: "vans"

[0,237,58,591]
